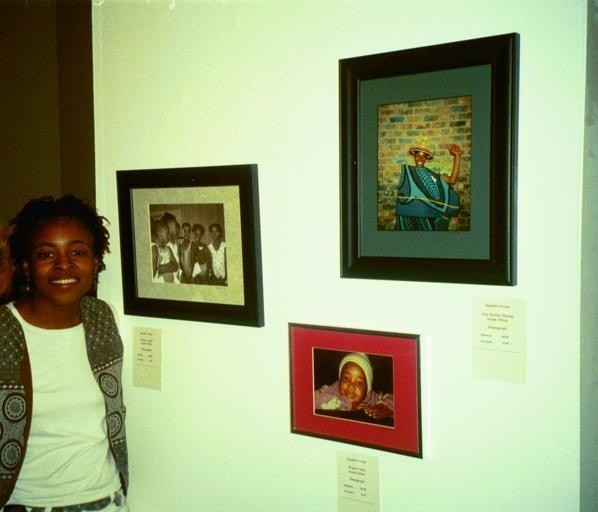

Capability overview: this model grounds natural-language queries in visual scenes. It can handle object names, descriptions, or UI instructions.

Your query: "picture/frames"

[337,29,522,287]
[286,318,425,461]
[115,162,265,333]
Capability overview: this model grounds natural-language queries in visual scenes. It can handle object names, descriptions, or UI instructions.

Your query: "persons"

[151,212,227,284]
[316,352,394,422]
[394,143,462,231]
[0,195,130,512]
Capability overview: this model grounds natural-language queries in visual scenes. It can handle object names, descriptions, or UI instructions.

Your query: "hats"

[408,145,434,160]
[339,352,374,393]
